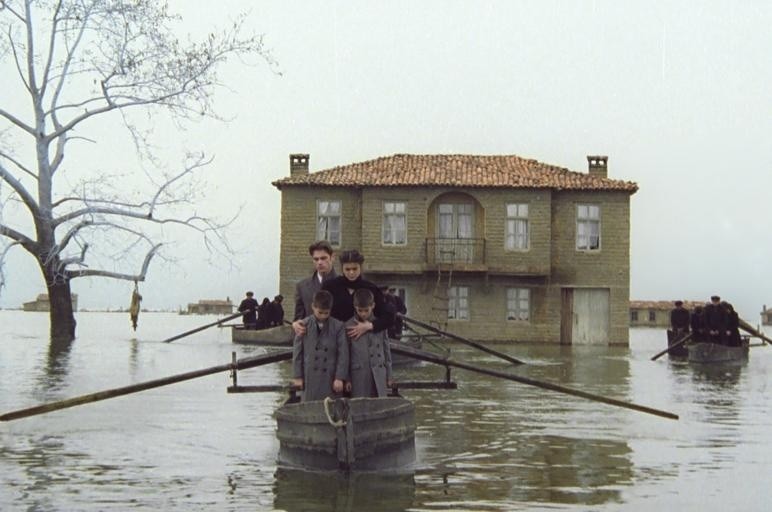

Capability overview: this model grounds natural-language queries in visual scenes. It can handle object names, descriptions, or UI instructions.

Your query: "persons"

[670,295,742,347]
[239,291,284,330]
[291,239,407,403]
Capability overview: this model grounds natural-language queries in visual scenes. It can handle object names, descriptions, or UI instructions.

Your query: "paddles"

[1,350,294,426]
[651,332,691,362]
[161,304,259,343]
[739,322,772,345]
[397,311,523,365]
[385,343,681,421]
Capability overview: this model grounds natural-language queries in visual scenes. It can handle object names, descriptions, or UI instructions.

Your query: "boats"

[231,324,422,474]
[666,329,750,364]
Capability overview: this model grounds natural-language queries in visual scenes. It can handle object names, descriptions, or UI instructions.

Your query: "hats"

[711,296,720,301]
[675,301,683,305]
[246,292,254,296]
[339,250,365,264]
